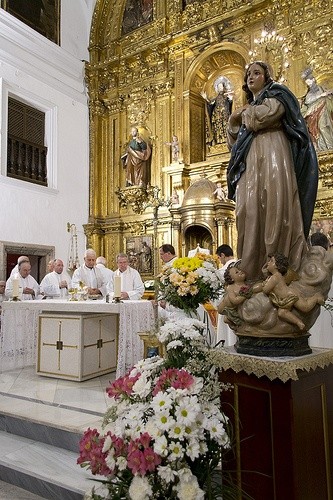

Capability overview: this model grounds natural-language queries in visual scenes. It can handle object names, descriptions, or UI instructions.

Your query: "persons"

[201,82,235,145]
[0,232,333,350]
[167,135,180,163]
[122,127,151,187]
[304,76,333,151]
[213,183,227,203]
[136,242,152,272]
[169,191,179,204]
[217,266,254,327]
[260,254,326,330]
[224,61,318,285]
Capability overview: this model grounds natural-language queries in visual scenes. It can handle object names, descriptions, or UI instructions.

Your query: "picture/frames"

[124,234,154,276]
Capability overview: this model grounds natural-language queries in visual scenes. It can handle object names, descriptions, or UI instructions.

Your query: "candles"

[13,273,18,297]
[114,268,121,298]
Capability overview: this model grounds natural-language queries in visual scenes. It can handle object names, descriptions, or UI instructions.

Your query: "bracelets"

[31,289,35,294]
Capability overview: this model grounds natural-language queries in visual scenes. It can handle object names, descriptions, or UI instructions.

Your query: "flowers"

[76,252,234,500]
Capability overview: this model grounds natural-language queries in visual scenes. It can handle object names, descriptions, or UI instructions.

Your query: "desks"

[0,298,155,379]
